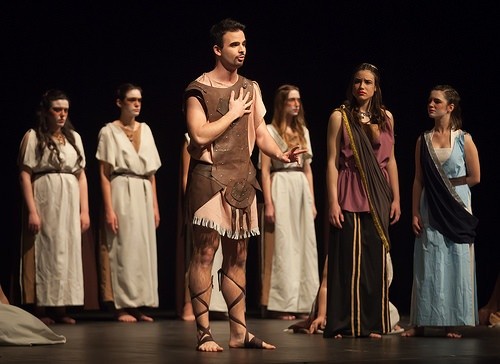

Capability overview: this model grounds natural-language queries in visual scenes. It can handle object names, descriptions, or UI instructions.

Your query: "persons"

[17,90,90,325]
[95,82,161,323]
[257,85,317,320]
[181,19,310,351]
[308,63,402,340]
[177,132,235,323]
[402,86,481,339]
